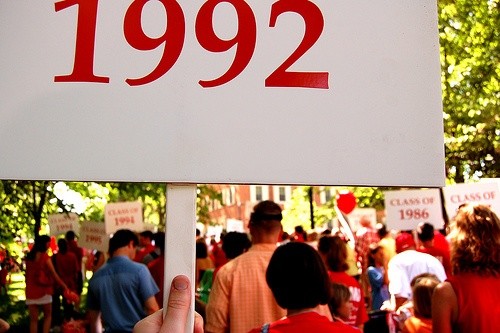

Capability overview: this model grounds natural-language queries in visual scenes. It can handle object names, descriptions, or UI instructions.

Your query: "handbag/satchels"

[39,271,54,285]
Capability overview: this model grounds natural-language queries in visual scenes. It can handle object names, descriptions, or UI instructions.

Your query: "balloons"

[336,191,358,214]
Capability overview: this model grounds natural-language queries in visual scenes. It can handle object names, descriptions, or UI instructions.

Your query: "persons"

[431,204,500,333]
[25,235,68,333]
[65,230,83,312]
[86,214,468,333]
[87,229,160,333]
[247,242,363,333]
[205,200,334,333]
[133,275,204,333]
[0,249,21,297]
[52,238,76,328]
